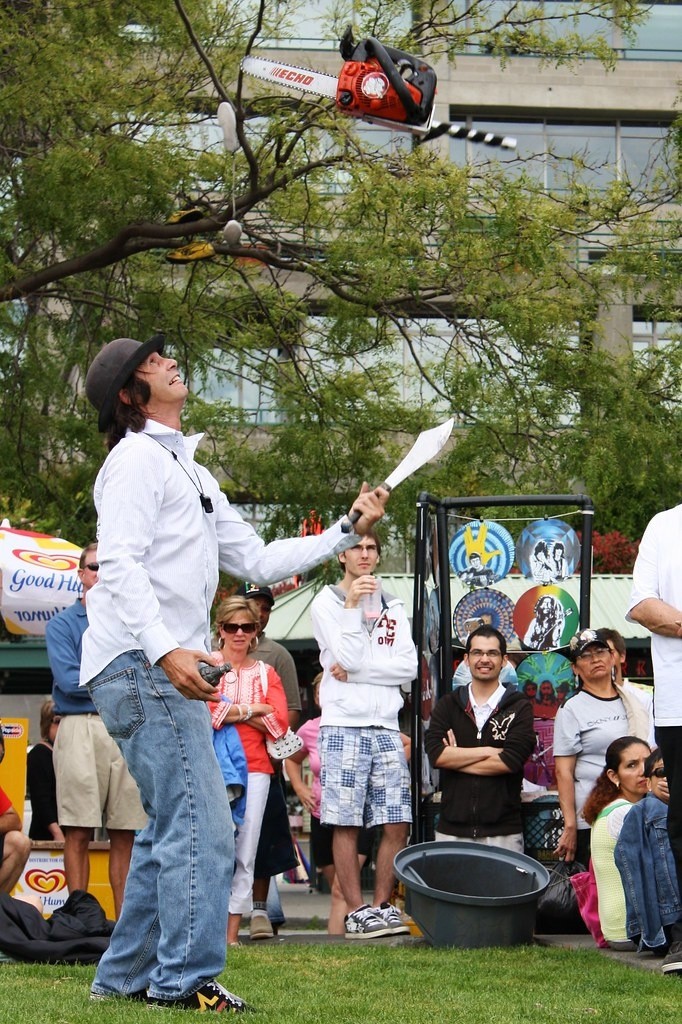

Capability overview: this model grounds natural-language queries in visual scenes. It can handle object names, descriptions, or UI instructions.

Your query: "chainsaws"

[242,24,517,152]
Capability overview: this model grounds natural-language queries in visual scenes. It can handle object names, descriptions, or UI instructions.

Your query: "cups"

[363,579,381,618]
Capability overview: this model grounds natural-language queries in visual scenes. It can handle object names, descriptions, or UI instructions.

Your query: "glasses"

[578,648,610,660]
[81,563,99,571]
[49,716,62,724]
[650,767,666,778]
[221,622,256,633]
[468,650,503,658]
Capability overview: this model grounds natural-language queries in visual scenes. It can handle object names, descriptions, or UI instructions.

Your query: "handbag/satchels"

[264,726,305,760]
[535,857,587,935]
[570,856,609,948]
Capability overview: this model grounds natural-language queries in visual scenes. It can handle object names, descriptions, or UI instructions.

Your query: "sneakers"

[249,915,273,938]
[89,989,147,1004]
[373,903,410,933]
[344,905,392,939]
[146,980,258,1015]
[662,941,682,972]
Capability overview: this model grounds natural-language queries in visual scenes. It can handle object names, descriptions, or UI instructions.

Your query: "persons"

[0,700,68,932]
[553,627,682,956]
[78,338,389,1014]
[204,526,417,946]
[45,543,150,924]
[423,624,537,855]
[624,505,682,974]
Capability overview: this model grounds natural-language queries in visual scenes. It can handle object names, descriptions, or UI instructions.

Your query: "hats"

[85,335,164,435]
[235,582,274,606]
[569,628,609,662]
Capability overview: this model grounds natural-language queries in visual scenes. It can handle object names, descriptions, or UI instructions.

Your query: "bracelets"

[243,703,252,721]
[235,704,242,722]
